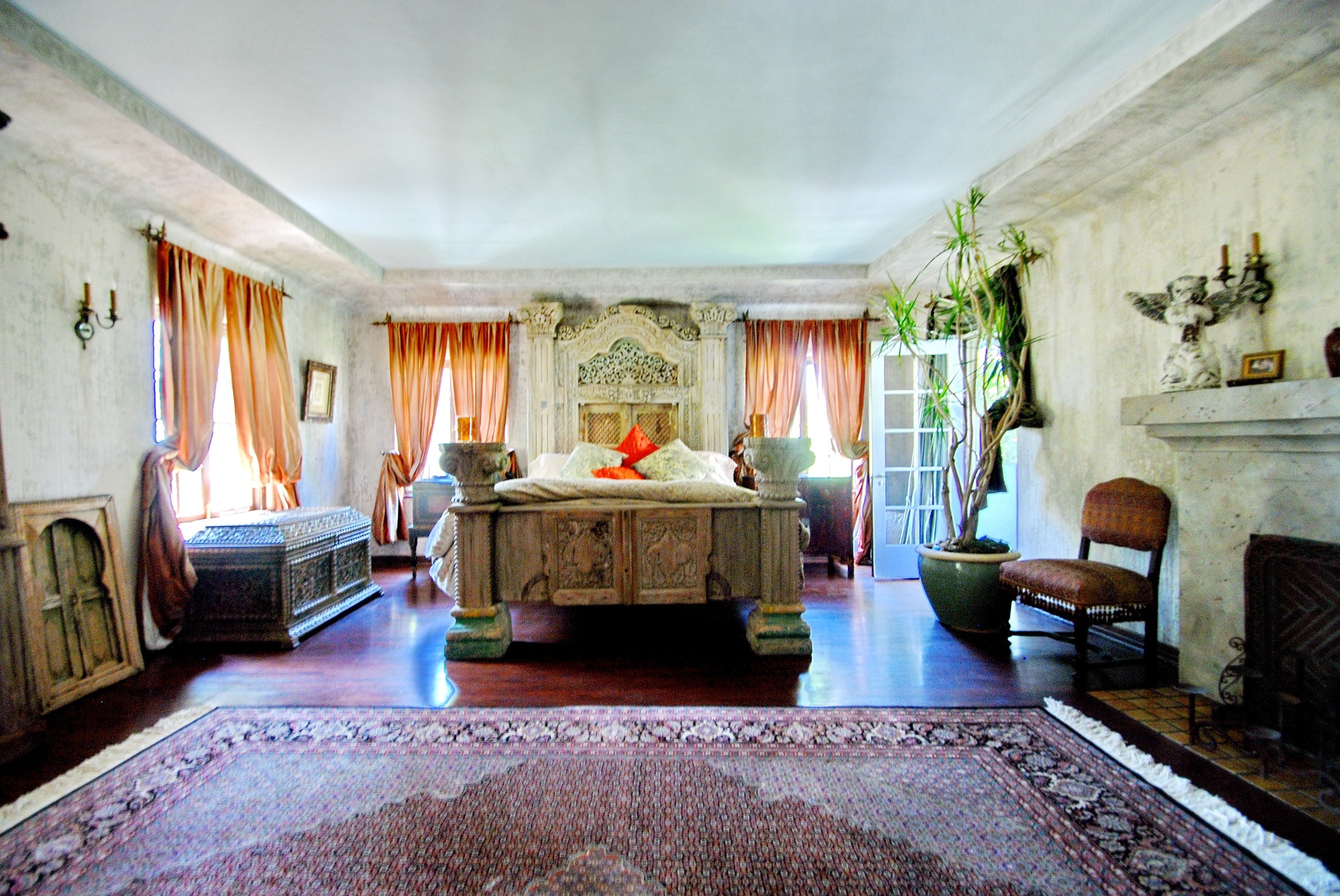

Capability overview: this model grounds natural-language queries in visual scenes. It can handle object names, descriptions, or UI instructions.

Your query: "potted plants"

[874,185,1046,632]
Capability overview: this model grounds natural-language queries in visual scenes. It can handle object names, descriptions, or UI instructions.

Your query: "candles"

[111,290,116,311]
[1222,245,1228,266]
[1252,232,1260,253]
[84,283,90,307]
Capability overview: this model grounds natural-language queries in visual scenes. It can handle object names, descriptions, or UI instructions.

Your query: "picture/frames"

[1244,349,1283,379]
[298,360,337,425]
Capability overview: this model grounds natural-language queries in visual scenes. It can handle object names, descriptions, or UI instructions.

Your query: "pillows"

[560,424,709,483]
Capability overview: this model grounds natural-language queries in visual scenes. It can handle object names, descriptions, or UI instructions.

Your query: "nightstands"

[793,476,854,581]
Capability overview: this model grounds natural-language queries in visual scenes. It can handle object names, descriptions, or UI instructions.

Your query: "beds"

[439,421,815,663]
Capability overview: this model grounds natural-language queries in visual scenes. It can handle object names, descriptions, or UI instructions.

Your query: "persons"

[1160,275,1221,394]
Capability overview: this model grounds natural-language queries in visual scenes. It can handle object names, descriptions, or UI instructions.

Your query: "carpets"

[0,697,1340,896]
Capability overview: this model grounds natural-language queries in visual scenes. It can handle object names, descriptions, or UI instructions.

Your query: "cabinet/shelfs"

[180,506,382,652]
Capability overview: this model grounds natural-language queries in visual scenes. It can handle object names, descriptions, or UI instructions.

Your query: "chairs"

[997,476,1173,687]
[408,482,456,583]
